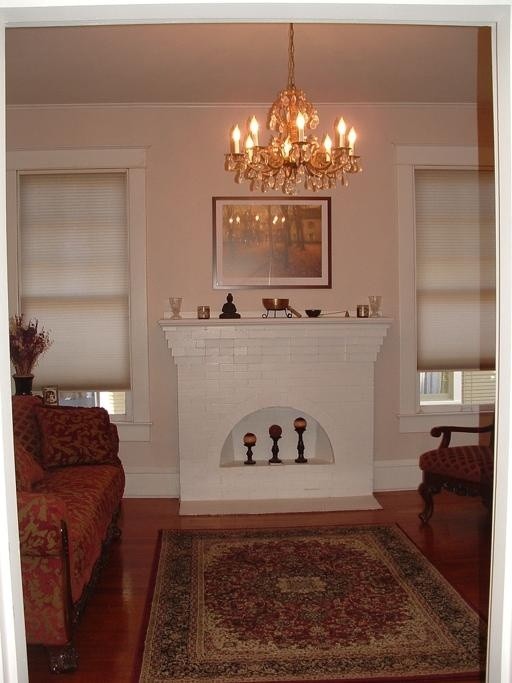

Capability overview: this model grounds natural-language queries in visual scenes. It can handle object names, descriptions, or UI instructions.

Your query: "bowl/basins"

[305,309,322,317]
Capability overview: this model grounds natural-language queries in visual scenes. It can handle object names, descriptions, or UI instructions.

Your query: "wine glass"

[370,295,381,318]
[169,296,184,320]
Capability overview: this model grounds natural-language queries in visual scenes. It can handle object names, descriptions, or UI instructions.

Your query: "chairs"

[419,424,494,523]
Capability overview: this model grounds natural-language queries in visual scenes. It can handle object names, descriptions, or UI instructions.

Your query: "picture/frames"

[213,197,331,289]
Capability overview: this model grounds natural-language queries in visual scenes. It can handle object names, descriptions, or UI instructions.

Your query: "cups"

[197,305,210,318]
[357,305,369,318]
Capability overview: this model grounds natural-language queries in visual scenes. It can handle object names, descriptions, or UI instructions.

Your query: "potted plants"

[9,313,54,395]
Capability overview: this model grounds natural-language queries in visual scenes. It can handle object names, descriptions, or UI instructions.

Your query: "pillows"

[15,436,44,488]
[35,405,114,469]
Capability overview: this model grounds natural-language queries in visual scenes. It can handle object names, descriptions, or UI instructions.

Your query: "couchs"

[13,396,125,674]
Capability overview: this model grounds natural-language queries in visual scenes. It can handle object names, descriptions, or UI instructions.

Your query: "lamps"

[224,24,360,195]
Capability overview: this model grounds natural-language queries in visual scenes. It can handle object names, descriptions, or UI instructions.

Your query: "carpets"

[134,522,488,682]
